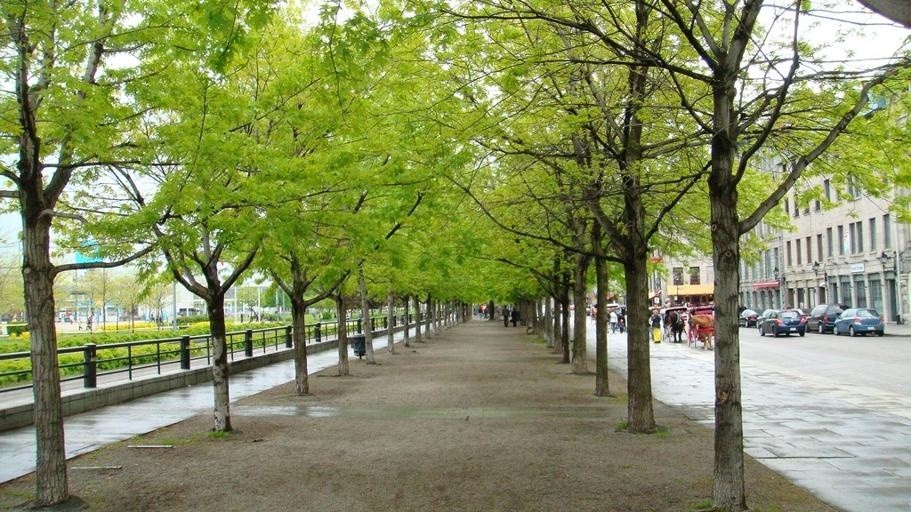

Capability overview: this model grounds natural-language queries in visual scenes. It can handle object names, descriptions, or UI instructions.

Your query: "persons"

[477,305,489,319]
[501,306,510,328]
[651,310,662,343]
[511,307,518,328]
[606,307,625,334]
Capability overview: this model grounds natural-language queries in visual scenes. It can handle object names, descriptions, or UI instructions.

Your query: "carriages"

[580,299,715,352]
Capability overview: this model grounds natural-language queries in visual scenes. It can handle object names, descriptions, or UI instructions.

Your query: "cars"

[833,307,884,335]
[739,309,764,327]
[759,309,806,337]
[789,308,810,323]
[806,303,849,334]
[754,309,776,328]
